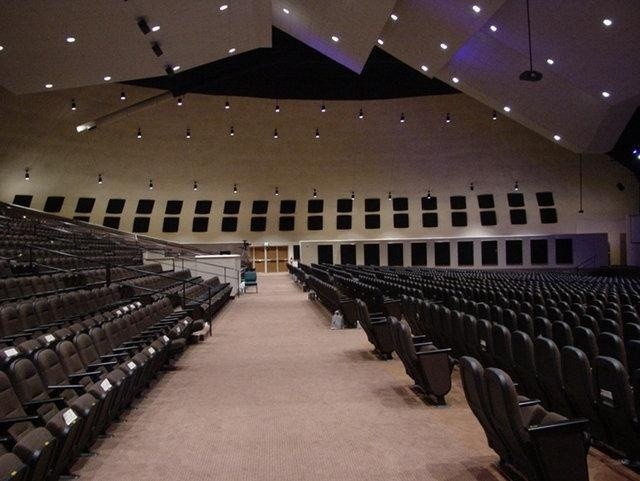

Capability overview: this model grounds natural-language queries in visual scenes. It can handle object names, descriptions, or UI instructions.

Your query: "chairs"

[0,202,232,481]
[244,271,258,292]
[286,261,640,481]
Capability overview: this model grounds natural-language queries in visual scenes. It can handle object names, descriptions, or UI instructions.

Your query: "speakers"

[616,183,624,191]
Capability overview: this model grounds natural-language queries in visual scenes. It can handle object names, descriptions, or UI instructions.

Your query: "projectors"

[519,70,544,83]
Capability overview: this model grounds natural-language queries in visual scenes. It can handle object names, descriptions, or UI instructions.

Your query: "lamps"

[223,101,232,114]
[313,129,322,143]
[136,15,152,35]
[27,171,518,200]
[165,64,175,76]
[274,103,281,114]
[319,103,328,118]
[184,127,193,144]
[134,130,145,141]
[69,100,78,113]
[443,112,455,128]
[489,109,498,123]
[397,112,407,125]
[271,129,280,141]
[118,91,128,104]
[355,109,367,121]
[228,128,236,140]
[151,40,163,57]
[174,97,185,110]
